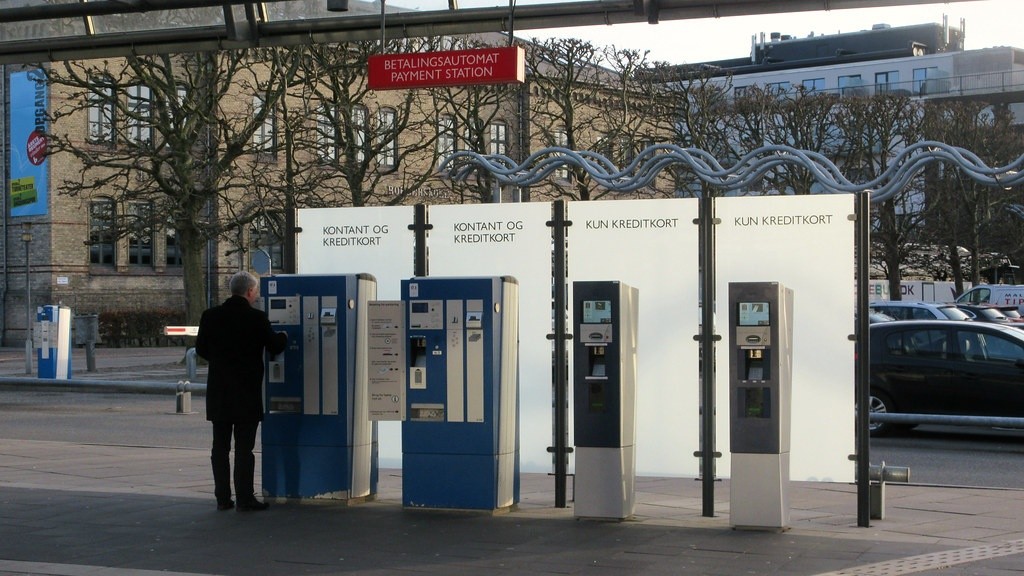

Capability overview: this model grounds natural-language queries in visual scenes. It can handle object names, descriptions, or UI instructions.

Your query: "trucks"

[955,283,1024,316]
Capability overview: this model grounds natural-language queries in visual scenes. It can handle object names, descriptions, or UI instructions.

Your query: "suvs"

[855,301,1024,437]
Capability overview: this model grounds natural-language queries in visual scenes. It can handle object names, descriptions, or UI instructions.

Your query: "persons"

[196,271,288,510]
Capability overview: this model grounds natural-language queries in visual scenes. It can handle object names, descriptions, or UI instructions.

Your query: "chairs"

[935,339,947,359]
[960,337,969,351]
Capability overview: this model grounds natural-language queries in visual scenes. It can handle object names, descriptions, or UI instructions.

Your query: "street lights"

[21,222,32,375]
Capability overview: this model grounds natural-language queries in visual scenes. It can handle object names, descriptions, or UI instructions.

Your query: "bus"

[854,243,1022,284]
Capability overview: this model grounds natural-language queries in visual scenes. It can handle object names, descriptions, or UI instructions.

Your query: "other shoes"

[215,494,235,511]
[237,494,270,511]
